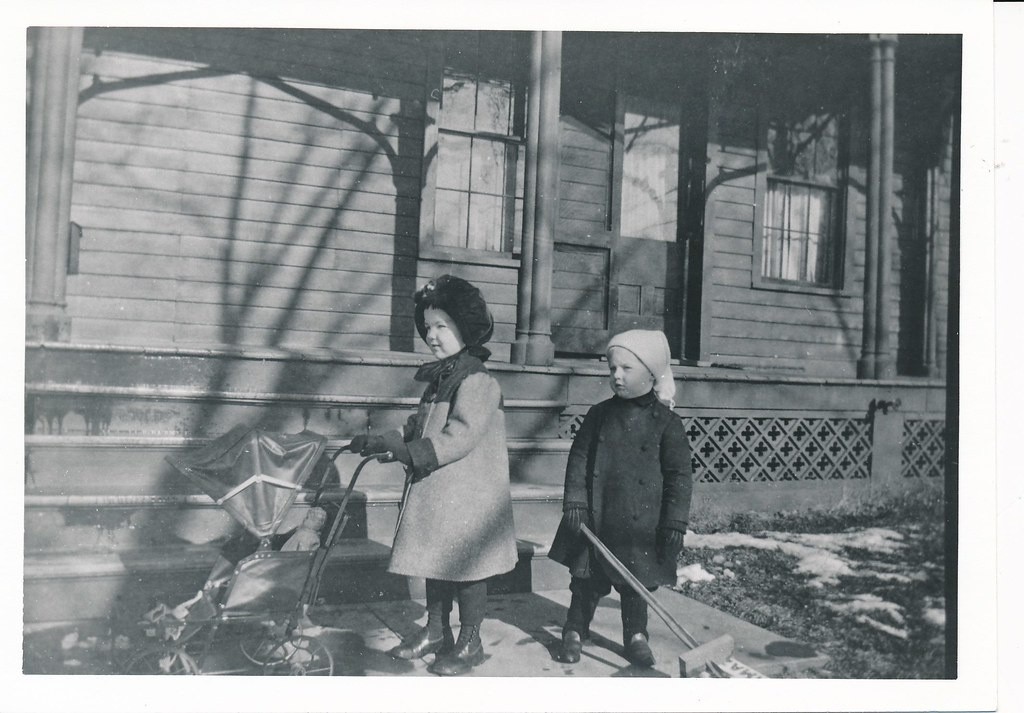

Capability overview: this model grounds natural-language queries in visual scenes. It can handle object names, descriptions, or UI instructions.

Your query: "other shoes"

[561,630,582,662]
[624,632,656,664]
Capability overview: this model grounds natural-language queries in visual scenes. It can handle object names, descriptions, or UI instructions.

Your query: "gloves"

[350,428,402,453]
[360,441,412,465]
[563,509,588,538]
[659,528,684,560]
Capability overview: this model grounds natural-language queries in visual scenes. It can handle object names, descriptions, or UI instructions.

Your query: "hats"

[414,274,494,362]
[606,328,676,410]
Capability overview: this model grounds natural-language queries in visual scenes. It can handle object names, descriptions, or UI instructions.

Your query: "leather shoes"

[433,640,485,675]
[392,625,455,659]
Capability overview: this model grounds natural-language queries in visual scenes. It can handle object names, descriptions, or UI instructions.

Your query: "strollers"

[121,426,397,675]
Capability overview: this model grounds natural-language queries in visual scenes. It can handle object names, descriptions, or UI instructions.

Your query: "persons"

[547,329,693,665]
[349,274,519,674]
[280,504,327,552]
